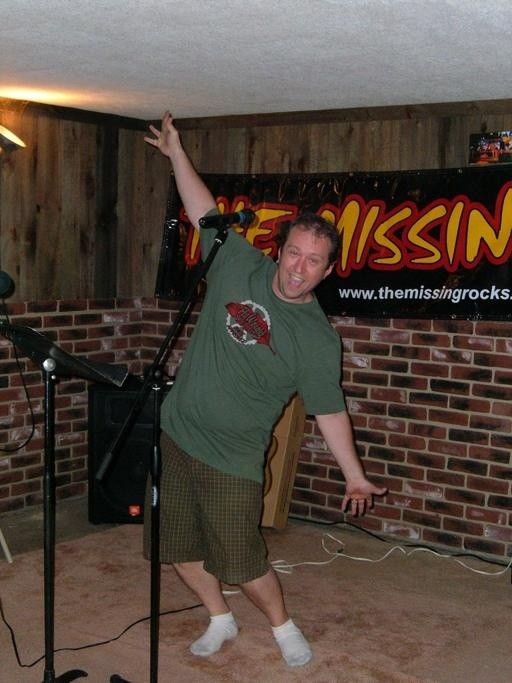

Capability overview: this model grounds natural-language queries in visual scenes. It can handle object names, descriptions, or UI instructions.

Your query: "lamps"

[1,121,27,163]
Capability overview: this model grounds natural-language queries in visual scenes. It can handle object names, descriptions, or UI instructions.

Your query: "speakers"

[87,376,174,525]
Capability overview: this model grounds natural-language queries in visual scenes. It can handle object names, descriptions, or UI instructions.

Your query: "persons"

[142,109,388,669]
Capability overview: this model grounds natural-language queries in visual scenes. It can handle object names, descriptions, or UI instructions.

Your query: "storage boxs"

[259,393,305,533]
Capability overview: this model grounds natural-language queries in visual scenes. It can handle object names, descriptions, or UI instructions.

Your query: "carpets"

[0,515,512,680]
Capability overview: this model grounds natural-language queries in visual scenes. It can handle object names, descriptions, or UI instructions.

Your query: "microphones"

[199,208,256,230]
